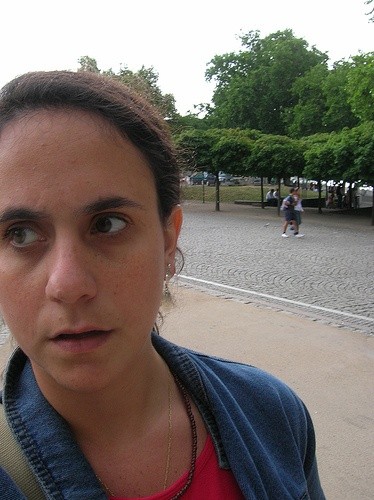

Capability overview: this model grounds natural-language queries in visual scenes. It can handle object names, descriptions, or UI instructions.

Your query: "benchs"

[235,193,329,213]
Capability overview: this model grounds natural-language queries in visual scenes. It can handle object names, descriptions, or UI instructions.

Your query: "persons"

[0,69,326,499]
[306,182,357,210]
[274,189,280,205]
[266,189,276,206]
[280,188,304,237]
[290,187,303,233]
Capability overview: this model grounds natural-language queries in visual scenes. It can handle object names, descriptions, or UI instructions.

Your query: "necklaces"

[95,383,172,498]
[93,371,198,500]
